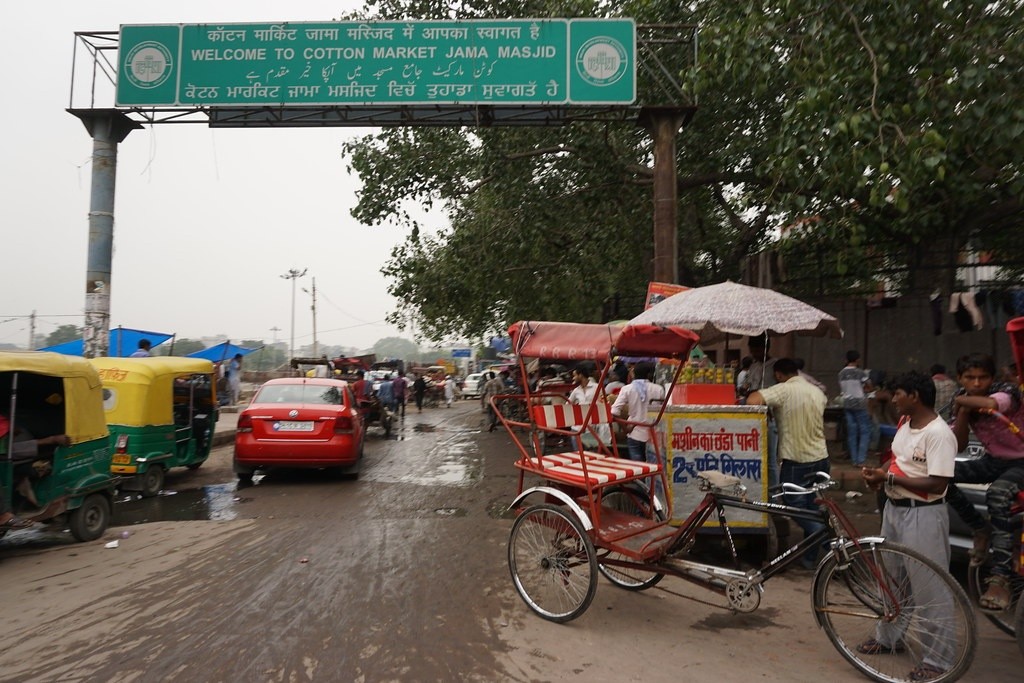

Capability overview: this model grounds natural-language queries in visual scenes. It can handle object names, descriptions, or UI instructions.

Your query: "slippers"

[856,639,905,655]
[906,662,947,682]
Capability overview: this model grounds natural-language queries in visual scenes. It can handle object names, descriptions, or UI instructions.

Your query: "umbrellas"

[615,280,844,367]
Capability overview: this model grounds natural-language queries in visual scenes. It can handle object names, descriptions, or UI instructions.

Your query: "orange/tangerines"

[674,365,734,384]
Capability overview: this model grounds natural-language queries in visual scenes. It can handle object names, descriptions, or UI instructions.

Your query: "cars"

[874,440,994,578]
[460,372,491,400]
[231,377,375,483]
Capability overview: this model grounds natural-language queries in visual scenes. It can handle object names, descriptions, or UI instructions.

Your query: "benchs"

[174,425,190,439]
[491,394,662,490]
[541,381,578,392]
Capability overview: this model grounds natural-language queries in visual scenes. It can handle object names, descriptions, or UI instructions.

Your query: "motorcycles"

[0,348,138,542]
[86,354,221,498]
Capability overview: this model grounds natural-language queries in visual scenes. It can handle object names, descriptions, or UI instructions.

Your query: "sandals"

[968,526,991,566]
[977,576,1014,614]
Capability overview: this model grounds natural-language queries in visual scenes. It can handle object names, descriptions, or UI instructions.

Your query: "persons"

[476,360,665,491]
[225,353,243,405]
[730,335,1024,683]
[0,434,73,531]
[129,339,153,357]
[314,354,456,417]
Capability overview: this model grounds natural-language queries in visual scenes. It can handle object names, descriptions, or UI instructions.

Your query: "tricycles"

[288,351,529,438]
[965,316,1024,665]
[487,279,980,683]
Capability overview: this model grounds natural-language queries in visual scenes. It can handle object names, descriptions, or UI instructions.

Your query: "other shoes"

[487,423,498,434]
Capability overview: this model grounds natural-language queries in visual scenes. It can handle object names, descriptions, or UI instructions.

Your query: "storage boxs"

[825,423,847,457]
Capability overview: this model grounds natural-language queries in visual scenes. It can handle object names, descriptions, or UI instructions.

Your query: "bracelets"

[887,472,894,486]
